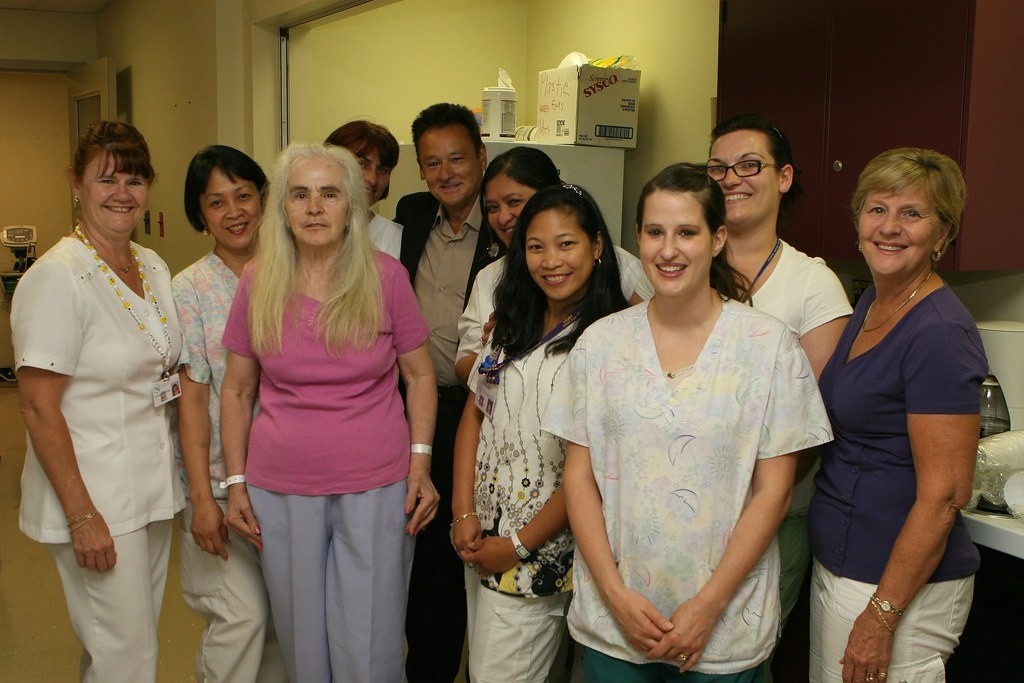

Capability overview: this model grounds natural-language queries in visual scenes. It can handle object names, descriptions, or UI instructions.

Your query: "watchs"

[511,532,531,559]
[872,591,905,616]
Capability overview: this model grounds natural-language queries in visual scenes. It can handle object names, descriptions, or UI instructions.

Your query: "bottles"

[479,87,517,141]
[979,369,1010,439]
[515,126,536,141]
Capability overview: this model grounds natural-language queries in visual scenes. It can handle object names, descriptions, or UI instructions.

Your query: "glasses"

[706,159,778,181]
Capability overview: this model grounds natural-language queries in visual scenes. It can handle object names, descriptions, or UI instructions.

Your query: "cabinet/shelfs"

[712,0,1024,277]
[377,143,625,248]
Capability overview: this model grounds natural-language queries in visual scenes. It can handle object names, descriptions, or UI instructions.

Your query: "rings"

[480,335,486,344]
[877,671,888,679]
[865,672,877,682]
[679,653,688,662]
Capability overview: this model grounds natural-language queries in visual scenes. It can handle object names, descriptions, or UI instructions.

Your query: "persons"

[9,119,181,683]
[171,103,654,683]
[707,117,855,683]
[173,384,180,396]
[807,148,987,683]
[537,163,834,683]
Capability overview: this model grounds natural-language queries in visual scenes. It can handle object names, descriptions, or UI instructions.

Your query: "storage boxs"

[535,64,641,150]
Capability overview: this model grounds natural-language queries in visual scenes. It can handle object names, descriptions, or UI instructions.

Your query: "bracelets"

[410,443,432,456]
[450,512,478,527]
[869,597,896,636]
[68,507,98,534]
[220,474,246,490]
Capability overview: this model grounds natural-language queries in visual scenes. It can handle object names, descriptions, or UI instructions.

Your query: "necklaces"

[860,272,932,332]
[100,250,136,274]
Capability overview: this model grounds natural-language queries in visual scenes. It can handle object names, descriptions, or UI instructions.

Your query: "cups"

[979,430,1024,515]
